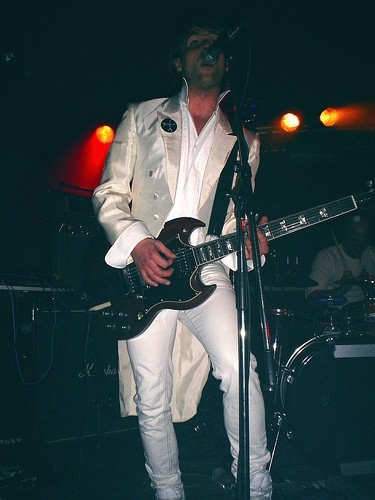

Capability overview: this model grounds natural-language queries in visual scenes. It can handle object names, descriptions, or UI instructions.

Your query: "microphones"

[200,24,241,66]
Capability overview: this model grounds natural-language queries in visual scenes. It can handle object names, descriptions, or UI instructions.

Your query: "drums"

[278,330,375,476]
[307,289,348,306]
[343,298,375,323]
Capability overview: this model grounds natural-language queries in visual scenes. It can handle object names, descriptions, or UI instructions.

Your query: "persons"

[92,15,276,500]
[305,209,375,307]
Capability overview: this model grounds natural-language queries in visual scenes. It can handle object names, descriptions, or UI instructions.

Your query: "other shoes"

[154,482,185,500]
[234,470,273,500]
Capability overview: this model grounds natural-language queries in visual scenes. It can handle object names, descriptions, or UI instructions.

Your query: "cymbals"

[254,269,321,288]
[333,274,374,286]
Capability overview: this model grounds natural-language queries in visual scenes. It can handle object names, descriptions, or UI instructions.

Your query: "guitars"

[70,179,375,341]
